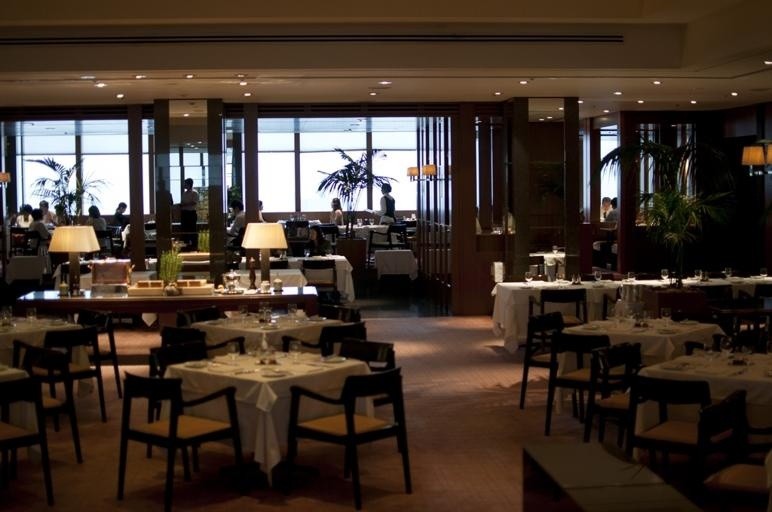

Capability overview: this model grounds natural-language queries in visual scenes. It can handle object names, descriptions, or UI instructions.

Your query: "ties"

[603,211,607,219]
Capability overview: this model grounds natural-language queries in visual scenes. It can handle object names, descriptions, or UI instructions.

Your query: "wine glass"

[525,272,534,287]
[594,270,602,285]
[760,267,767,280]
[628,272,636,285]
[303,249,310,259]
[661,306,673,330]
[552,245,558,256]
[370,218,374,226]
[719,336,733,363]
[724,266,732,281]
[661,269,669,283]
[288,340,303,365]
[290,215,295,222]
[694,269,703,285]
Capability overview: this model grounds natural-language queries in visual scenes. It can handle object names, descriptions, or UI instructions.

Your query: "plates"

[260,325,280,329]
[223,310,247,319]
[306,316,326,321]
[261,369,286,378]
[320,355,346,363]
[185,360,208,368]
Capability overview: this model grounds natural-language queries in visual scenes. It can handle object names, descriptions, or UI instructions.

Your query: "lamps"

[1,172,11,188]
[407,167,425,182]
[741,147,765,176]
[241,222,289,282]
[48,225,100,295]
[423,164,438,182]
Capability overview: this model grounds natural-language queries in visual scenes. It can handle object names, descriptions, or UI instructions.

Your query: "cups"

[287,303,296,320]
[26,307,37,322]
[59,283,69,295]
[273,278,283,292]
[2,305,12,324]
[258,301,271,323]
[357,219,362,227]
[703,334,713,354]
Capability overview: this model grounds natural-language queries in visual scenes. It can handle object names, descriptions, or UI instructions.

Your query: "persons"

[226,200,246,269]
[258,200,266,223]
[15,205,33,256]
[177,178,199,251]
[156,180,173,238]
[39,200,54,228]
[323,198,344,242]
[604,198,617,222]
[601,197,614,222]
[113,203,130,248]
[371,184,396,225]
[28,209,68,274]
[84,206,106,274]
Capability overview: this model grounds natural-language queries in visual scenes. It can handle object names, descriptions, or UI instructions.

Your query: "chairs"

[368,224,409,262]
[281,321,367,356]
[528,289,587,327]
[23,229,49,254]
[624,374,733,463]
[302,259,337,290]
[520,312,579,418]
[1,377,56,506]
[22,351,84,464]
[75,308,123,398]
[148,348,200,421]
[288,366,412,511]
[339,338,395,406]
[695,389,768,509]
[44,326,107,433]
[582,342,648,444]
[160,326,246,358]
[116,370,242,511]
[602,286,622,320]
[96,228,113,249]
[544,330,629,443]
